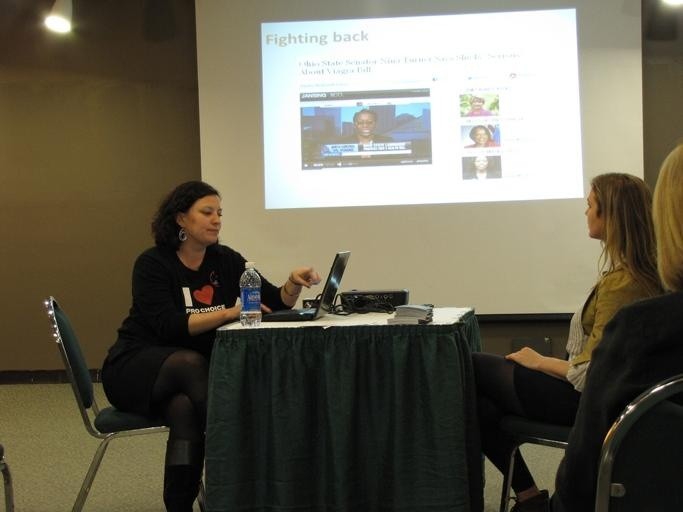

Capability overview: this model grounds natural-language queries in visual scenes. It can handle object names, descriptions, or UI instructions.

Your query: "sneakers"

[509,488,551,512]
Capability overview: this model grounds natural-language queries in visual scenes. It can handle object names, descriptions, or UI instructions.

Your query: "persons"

[463,125,499,147]
[466,97,492,117]
[551,143,683,511]
[101,181,321,511]
[472,172,663,511]
[465,157,499,179]
[336,109,404,165]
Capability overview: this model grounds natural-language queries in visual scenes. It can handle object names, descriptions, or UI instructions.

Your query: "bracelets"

[282,284,300,298]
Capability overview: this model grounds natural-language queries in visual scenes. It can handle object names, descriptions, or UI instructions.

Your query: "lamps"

[43,0,72,34]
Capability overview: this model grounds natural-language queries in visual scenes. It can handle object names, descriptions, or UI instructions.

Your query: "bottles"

[238,261,263,328]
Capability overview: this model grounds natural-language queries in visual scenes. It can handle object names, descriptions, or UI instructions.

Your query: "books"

[387,303,434,325]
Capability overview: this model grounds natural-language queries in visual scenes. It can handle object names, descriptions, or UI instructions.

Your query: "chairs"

[595,375,683,512]
[43,295,206,511]
[500,413,573,511]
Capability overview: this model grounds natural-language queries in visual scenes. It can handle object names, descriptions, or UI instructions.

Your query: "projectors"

[339,289,409,313]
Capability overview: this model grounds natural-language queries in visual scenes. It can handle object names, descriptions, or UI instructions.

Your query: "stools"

[0,445,15,512]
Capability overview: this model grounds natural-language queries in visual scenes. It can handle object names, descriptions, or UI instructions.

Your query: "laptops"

[261,250,351,322]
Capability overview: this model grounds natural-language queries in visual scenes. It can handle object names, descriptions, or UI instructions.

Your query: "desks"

[207,306,482,511]
